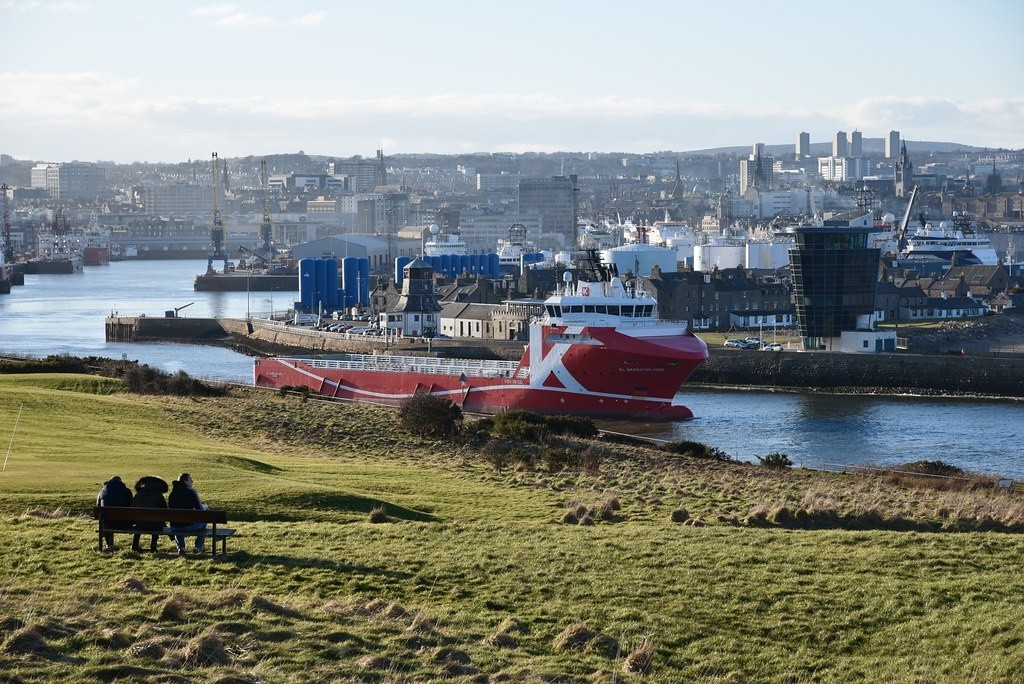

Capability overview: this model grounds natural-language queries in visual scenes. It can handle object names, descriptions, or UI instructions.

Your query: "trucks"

[297,314,318,323]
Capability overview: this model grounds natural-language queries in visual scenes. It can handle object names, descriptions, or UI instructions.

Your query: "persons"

[128,476,168,552]
[95,477,142,551]
[167,474,206,554]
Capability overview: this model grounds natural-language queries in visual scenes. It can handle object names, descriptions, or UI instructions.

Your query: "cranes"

[257,159,272,253]
[207,152,230,273]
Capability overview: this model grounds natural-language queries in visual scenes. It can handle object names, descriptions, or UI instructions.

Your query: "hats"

[135,476,169,493]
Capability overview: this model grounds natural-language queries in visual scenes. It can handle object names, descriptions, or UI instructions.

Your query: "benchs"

[93,505,236,555]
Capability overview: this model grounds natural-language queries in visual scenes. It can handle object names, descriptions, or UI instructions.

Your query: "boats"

[882,209,1000,278]
[252,246,710,424]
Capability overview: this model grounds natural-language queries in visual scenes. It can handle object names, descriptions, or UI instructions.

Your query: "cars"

[723,339,746,348]
[739,336,768,351]
[763,343,784,352]
[323,311,385,336]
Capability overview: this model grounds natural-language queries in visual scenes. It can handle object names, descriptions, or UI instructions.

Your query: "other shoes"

[106,545,111,548]
[193,547,201,552]
[132,545,141,551]
[152,548,157,553]
[177,548,184,554]
